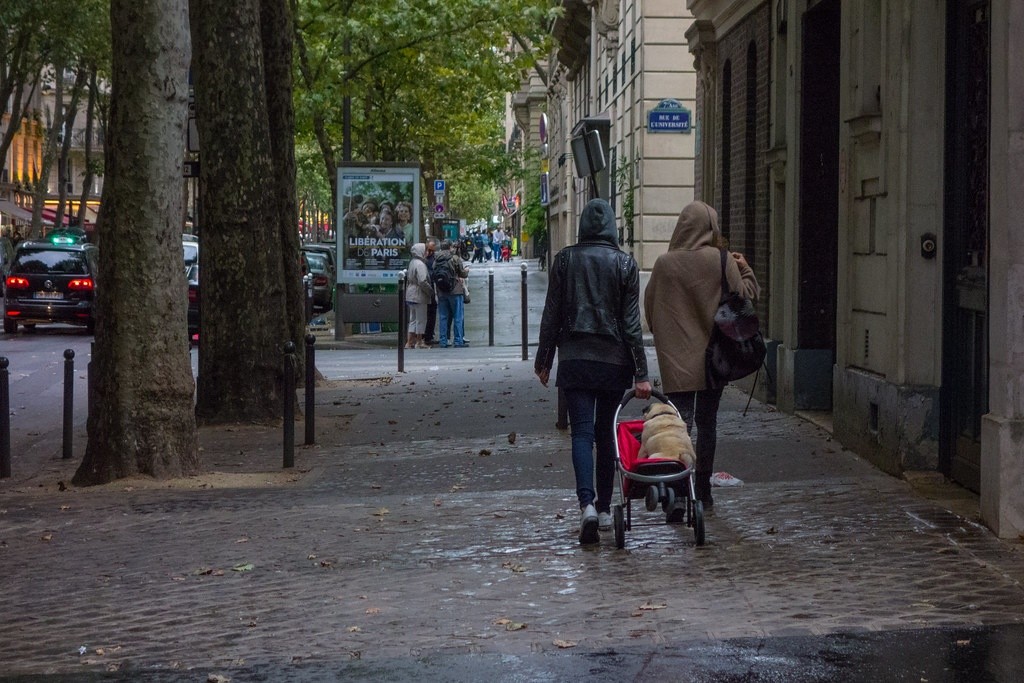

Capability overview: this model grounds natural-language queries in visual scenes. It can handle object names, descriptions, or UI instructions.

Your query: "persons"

[471,226,514,263]
[644,201,761,523]
[344,194,414,260]
[534,198,652,544]
[405,241,471,348]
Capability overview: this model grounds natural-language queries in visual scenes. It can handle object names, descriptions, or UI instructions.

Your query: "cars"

[302,243,335,318]
[181,240,200,335]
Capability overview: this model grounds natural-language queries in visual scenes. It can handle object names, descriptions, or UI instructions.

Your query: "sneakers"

[578,503,600,543]
[598,512,612,530]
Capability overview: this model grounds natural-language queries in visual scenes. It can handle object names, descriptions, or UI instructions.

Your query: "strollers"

[499,243,514,263]
[612,388,705,549]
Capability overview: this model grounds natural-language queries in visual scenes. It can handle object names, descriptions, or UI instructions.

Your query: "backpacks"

[432,251,456,292]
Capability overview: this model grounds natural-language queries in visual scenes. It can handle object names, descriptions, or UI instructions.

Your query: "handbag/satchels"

[705,247,768,390]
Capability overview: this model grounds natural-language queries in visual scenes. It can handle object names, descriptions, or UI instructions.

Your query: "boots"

[694,475,714,508]
[665,497,687,522]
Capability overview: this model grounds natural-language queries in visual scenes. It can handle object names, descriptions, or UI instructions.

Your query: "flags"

[502,194,510,214]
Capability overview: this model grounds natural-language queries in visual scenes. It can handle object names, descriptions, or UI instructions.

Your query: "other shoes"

[415,342,432,348]
[446,339,453,345]
[463,337,471,342]
[404,343,415,349]
[454,343,470,347]
[423,338,440,344]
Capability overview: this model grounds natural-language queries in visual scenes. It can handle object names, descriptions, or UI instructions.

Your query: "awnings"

[0,199,100,231]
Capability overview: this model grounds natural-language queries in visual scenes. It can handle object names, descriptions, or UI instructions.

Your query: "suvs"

[3,227,100,334]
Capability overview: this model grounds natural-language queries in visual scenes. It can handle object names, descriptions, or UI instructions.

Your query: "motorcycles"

[452,238,470,261]
